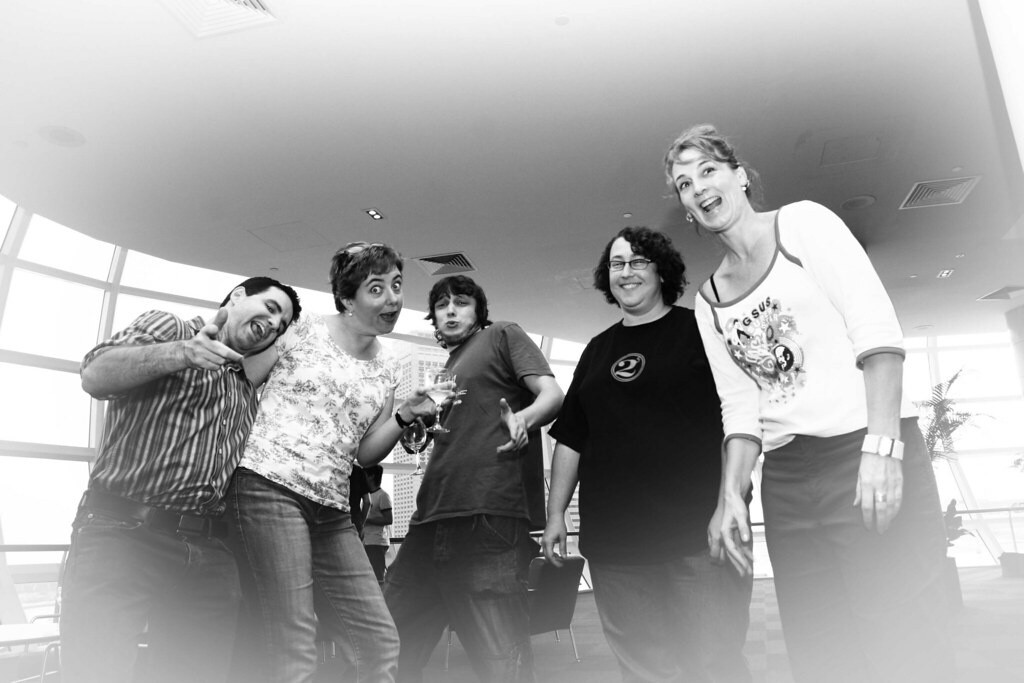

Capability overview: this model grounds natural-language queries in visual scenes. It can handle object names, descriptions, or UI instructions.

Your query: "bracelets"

[395,409,414,428]
[861,434,904,460]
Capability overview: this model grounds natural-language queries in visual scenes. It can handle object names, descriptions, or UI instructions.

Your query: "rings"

[876,497,887,502]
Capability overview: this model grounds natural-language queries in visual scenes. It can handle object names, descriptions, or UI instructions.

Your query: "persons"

[60,277,301,683]
[382,275,565,683]
[540,227,753,683]
[349,464,392,580]
[665,126,976,683]
[224,240,455,683]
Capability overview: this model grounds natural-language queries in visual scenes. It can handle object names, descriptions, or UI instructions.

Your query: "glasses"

[605,258,655,270]
[342,242,388,257]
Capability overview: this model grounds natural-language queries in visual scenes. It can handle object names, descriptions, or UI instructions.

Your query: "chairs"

[523,555,585,662]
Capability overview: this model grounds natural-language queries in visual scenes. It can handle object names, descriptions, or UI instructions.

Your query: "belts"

[85,492,219,540]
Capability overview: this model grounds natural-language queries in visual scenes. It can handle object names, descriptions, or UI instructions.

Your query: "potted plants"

[943,499,974,610]
